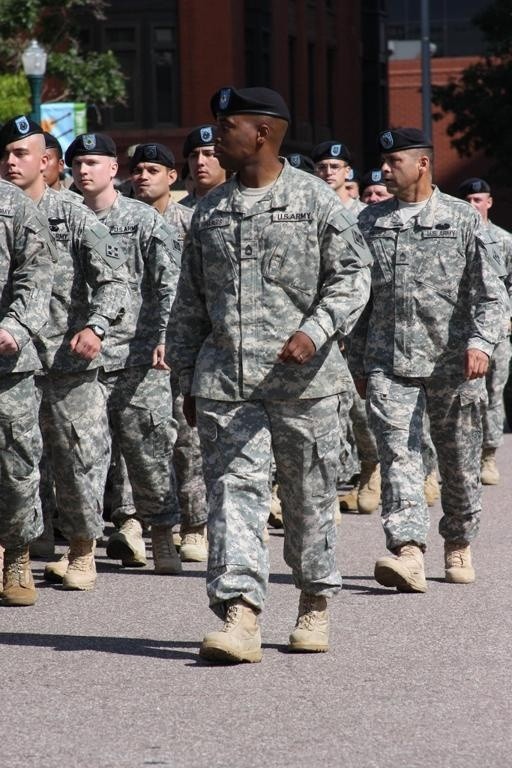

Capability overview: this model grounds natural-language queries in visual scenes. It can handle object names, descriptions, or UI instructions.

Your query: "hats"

[362,168,387,190]
[311,141,352,166]
[455,178,491,200]
[65,132,117,168]
[129,142,176,177]
[285,153,315,175]
[344,168,360,182]
[1,115,44,146]
[210,85,291,123]
[376,127,434,154]
[182,124,218,159]
[43,131,61,149]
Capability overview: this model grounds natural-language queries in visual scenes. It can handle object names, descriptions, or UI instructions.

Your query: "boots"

[424,466,440,508]
[179,525,208,562]
[1,544,39,604]
[443,542,476,583]
[480,448,500,485]
[62,538,97,592]
[356,461,382,514]
[105,517,148,568]
[289,591,331,652]
[339,479,361,512]
[43,547,70,584]
[199,598,263,663]
[374,544,428,594]
[30,521,56,558]
[150,525,183,573]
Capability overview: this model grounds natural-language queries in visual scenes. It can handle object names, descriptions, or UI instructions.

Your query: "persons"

[351,126,512,595]
[168,87,371,661]
[0,111,209,605]
